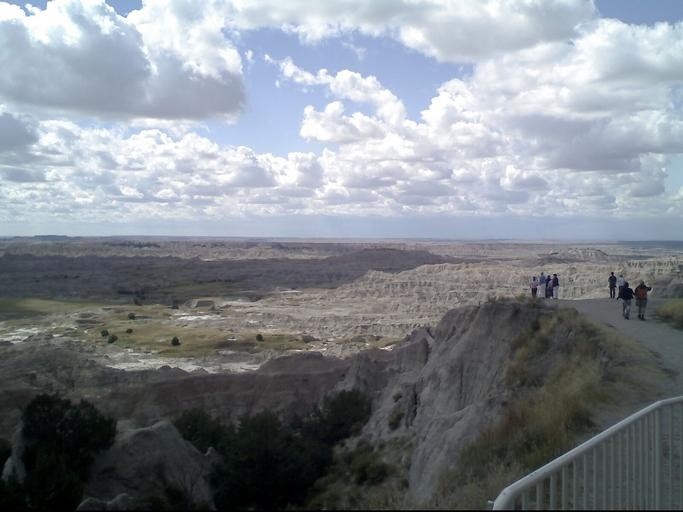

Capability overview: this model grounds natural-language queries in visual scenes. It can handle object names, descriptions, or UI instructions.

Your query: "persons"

[539,271,559,299]
[607,271,617,299]
[529,276,539,298]
[617,274,626,298]
[616,282,635,319]
[635,280,651,320]
